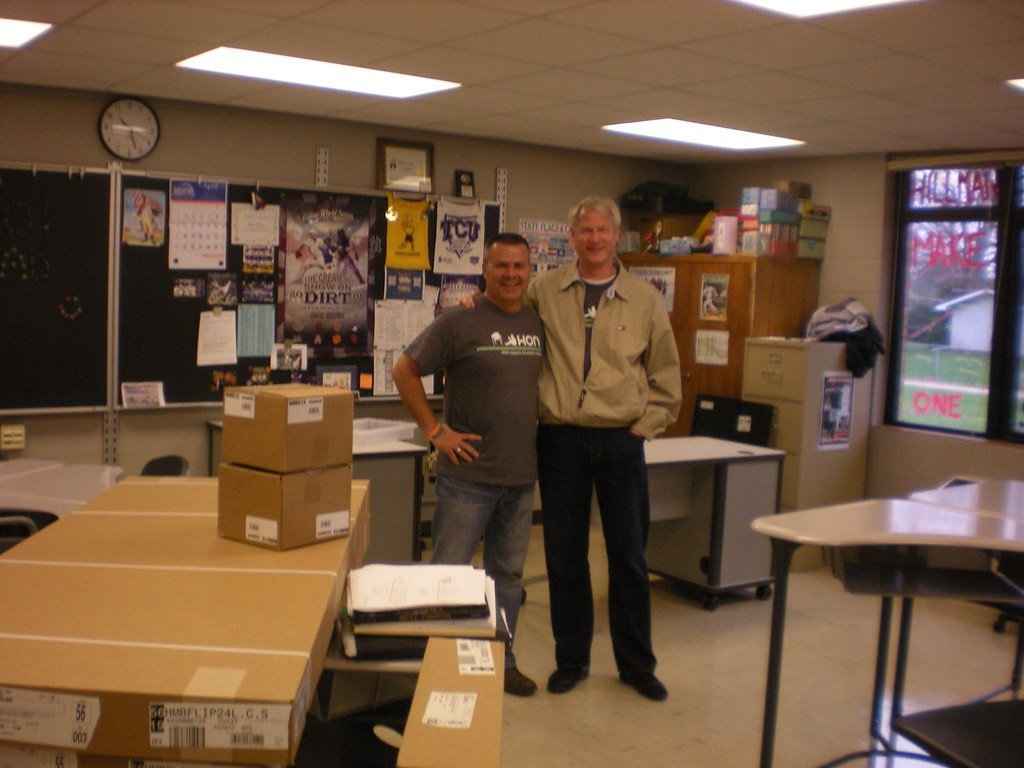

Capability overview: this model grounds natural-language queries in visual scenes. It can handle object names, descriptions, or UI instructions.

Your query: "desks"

[747,475,1024,768]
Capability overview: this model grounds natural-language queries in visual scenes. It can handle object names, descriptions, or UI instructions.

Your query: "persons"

[296,226,364,285]
[454,194,683,700]
[827,385,842,439]
[702,282,719,314]
[392,231,546,695]
[137,197,158,243]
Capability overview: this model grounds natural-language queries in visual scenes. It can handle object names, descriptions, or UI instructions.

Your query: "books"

[337,559,512,659]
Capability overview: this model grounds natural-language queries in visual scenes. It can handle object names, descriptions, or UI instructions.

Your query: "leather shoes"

[619,670,667,700]
[503,668,537,696]
[547,668,591,694]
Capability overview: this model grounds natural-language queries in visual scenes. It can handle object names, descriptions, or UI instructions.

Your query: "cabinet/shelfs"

[621,246,824,441]
[205,420,428,566]
[741,335,874,573]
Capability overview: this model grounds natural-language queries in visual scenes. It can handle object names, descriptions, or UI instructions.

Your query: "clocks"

[97,97,160,161]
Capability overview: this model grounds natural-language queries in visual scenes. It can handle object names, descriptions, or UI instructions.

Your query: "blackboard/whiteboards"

[0,159,116,414]
[114,168,502,411]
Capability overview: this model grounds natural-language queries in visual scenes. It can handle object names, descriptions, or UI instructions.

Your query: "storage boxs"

[1,382,509,767]
[630,177,833,260]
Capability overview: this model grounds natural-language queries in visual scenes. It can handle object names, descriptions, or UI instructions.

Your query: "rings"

[455,448,460,453]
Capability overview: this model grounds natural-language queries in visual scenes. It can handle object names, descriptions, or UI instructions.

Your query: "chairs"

[891,692,1023,767]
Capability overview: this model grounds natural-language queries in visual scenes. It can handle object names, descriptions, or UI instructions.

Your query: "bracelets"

[428,423,443,440]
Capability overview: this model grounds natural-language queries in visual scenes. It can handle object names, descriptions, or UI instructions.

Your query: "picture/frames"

[376,137,437,195]
